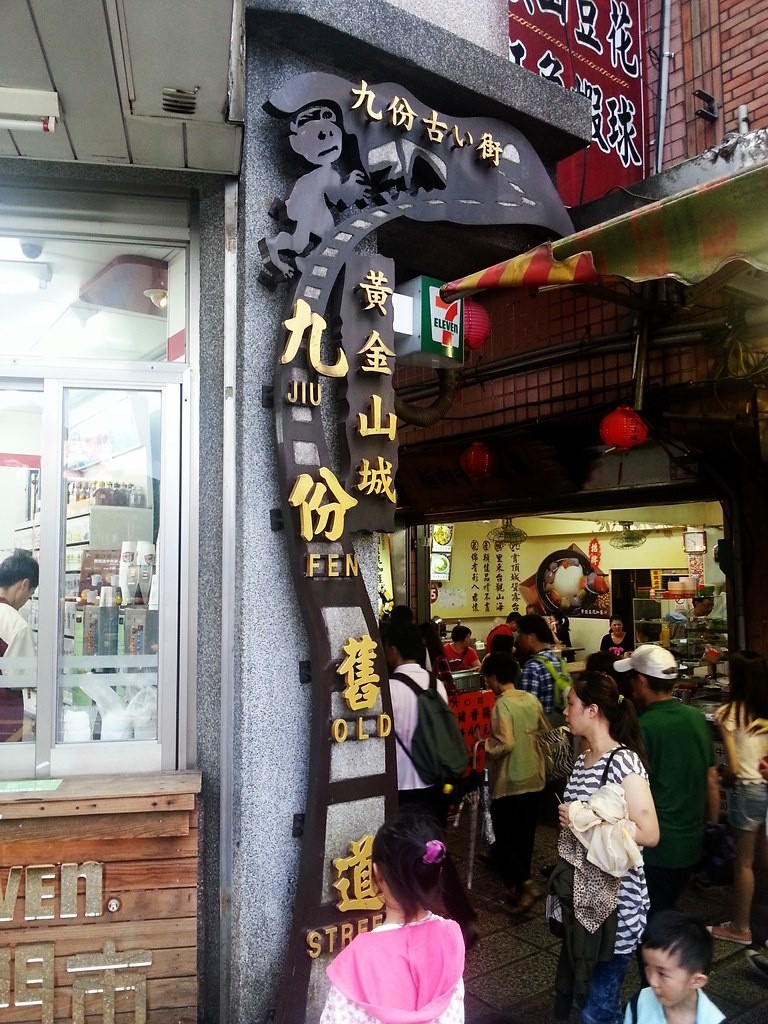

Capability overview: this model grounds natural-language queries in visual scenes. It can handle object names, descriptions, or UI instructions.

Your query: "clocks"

[682,531,707,553]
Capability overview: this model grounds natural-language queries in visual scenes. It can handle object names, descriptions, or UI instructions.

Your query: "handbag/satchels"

[537,726,574,779]
[545,862,577,937]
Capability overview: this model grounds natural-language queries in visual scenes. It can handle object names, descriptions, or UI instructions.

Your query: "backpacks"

[388,670,471,786]
[525,654,574,725]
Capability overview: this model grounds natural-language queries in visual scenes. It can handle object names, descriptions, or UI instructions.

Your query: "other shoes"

[464,922,479,950]
[509,880,544,917]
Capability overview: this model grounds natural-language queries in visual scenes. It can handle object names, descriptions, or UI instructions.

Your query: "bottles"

[661,626,670,648]
[65,522,89,596]
[649,587,656,599]
[67,479,145,508]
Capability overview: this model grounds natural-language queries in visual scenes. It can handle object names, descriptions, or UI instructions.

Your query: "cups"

[552,650,562,658]
[111,575,119,586]
[100,586,113,606]
[476,643,484,649]
[136,541,156,566]
[118,541,138,604]
[91,575,102,586]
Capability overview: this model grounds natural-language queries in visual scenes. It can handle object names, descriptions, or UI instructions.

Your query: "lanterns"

[459,441,490,477]
[597,405,648,452]
[464,300,490,349]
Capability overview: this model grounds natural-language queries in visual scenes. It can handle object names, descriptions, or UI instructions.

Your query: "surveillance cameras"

[18,239,45,260]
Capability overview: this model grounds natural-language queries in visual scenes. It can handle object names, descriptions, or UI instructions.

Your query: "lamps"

[0,88,59,133]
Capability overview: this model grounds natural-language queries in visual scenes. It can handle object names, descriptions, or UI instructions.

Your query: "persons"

[489,633,523,687]
[634,609,662,642]
[525,604,539,615]
[745,949,768,978]
[612,644,722,922]
[620,910,728,1024]
[552,672,662,1024]
[704,649,768,945]
[0,556,40,744]
[545,614,578,663]
[480,652,554,912]
[599,616,635,657]
[381,621,484,949]
[485,612,522,654]
[588,652,633,696]
[443,625,482,671]
[667,595,722,648]
[514,613,573,825]
[319,812,471,1024]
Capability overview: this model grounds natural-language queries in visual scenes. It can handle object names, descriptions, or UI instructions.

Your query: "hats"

[612,644,678,680]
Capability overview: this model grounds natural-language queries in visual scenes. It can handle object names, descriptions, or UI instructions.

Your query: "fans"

[487,518,527,546]
[609,521,646,549]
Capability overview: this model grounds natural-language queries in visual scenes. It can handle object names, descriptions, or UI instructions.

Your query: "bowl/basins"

[535,549,597,617]
[432,555,449,574]
[668,577,696,595]
[434,526,452,546]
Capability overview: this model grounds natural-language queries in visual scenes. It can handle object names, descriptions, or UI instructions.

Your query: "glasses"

[699,603,714,609]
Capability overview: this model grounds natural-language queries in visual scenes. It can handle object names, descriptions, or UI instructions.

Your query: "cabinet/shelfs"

[10,503,154,656]
[631,595,727,668]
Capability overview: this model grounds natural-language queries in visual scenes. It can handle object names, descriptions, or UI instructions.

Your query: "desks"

[557,647,588,677]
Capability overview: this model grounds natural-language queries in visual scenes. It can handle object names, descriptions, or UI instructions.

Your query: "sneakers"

[706,921,752,944]
[746,949,768,980]
